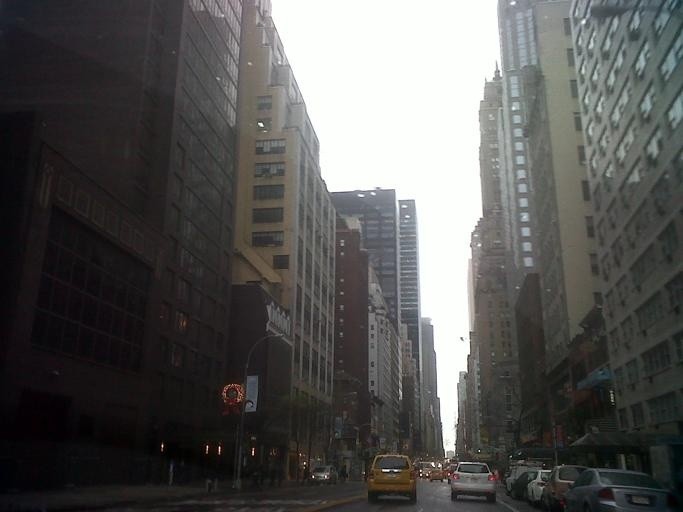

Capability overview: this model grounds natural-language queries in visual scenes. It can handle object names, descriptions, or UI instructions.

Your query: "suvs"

[539,465,588,511]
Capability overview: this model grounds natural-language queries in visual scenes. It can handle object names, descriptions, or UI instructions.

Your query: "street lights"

[237,332,283,477]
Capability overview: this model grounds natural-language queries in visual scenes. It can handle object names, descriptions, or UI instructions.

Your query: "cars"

[311,465,339,485]
[562,468,665,511]
[429,469,443,482]
[366,455,419,501]
[505,466,552,507]
[448,461,497,503]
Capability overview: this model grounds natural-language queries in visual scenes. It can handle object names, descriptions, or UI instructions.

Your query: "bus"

[415,461,443,479]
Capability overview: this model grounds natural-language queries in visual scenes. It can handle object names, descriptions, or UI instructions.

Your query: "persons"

[339,464,348,480]
[300,465,308,486]
